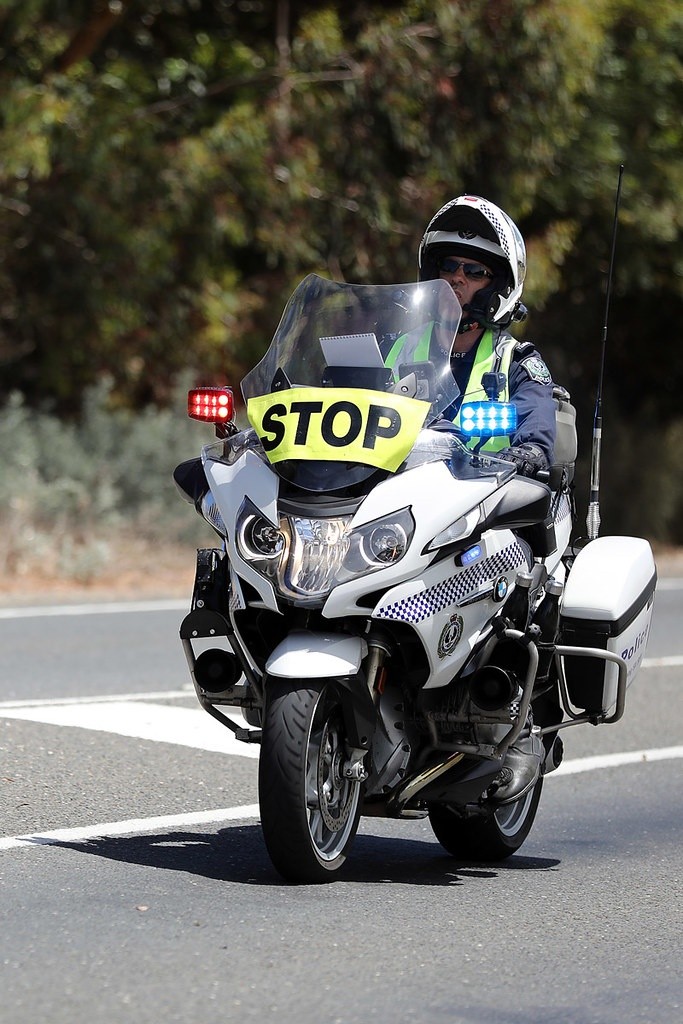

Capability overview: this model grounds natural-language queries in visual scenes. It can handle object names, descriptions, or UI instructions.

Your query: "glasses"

[435,257,495,283]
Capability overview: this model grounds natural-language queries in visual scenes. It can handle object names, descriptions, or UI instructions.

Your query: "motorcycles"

[172,163,657,887]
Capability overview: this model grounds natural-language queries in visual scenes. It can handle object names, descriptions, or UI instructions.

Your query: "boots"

[487,704,546,803]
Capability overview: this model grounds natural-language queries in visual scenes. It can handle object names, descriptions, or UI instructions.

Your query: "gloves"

[490,443,550,478]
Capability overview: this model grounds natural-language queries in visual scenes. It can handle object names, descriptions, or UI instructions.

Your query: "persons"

[377,196,579,803]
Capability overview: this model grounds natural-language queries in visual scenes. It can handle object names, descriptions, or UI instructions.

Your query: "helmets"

[419,195,526,325]
